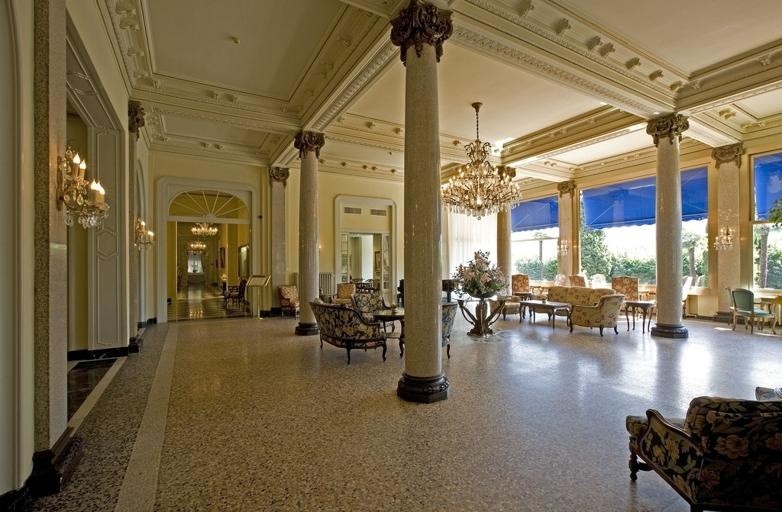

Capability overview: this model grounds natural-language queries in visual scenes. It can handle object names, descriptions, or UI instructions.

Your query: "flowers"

[452,249,511,302]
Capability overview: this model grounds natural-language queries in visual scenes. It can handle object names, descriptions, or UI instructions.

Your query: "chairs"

[529,273,782,340]
[227,278,247,310]
[279,272,528,365]
[627,377,776,511]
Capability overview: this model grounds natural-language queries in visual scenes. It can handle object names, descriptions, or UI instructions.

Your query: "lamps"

[190,242,208,256]
[714,221,735,252]
[56,148,108,232]
[190,223,222,237]
[441,101,525,221]
[135,217,154,254]
